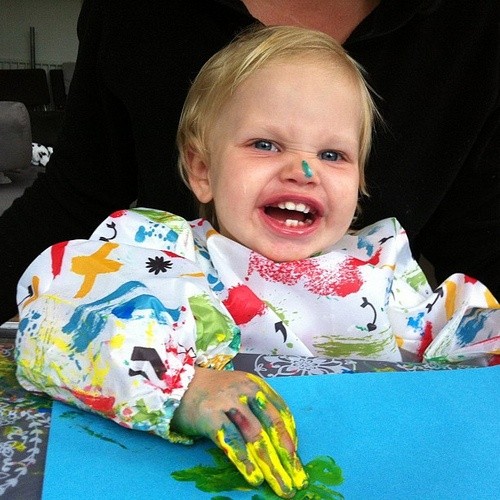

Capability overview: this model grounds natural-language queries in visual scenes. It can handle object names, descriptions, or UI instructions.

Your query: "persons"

[19,26,500,499]
[0,1,500,326]
